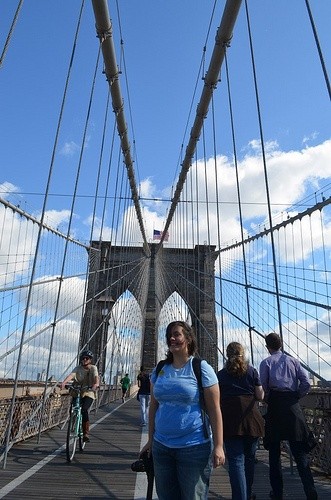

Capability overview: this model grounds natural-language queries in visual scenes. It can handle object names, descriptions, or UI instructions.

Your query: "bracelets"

[148,440,152,444]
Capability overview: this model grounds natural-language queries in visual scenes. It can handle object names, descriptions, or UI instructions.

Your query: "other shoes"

[84,436,90,442]
[248,490,256,500]
[269,489,279,499]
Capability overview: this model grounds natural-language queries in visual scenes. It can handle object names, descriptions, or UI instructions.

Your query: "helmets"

[79,349,94,360]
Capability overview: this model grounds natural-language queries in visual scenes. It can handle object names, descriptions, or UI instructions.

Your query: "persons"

[137,365,152,427]
[138,322,225,500]
[120,374,130,403]
[215,342,264,500]
[60,350,99,442]
[260,332,318,500]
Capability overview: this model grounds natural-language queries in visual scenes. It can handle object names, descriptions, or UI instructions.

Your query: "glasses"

[82,357,90,360]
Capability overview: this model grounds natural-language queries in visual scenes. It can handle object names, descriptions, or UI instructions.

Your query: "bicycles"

[60,385,100,463]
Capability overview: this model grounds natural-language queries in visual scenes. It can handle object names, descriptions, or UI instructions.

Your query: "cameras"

[131,451,154,474]
